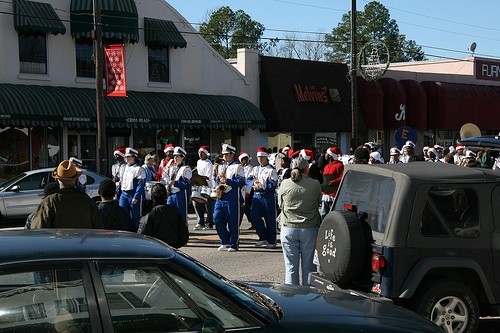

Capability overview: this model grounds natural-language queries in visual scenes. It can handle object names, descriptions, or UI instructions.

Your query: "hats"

[113,148,125,157]
[423,143,476,159]
[51,159,81,181]
[222,144,236,154]
[239,152,252,163]
[257,147,271,158]
[198,145,210,156]
[124,148,138,157]
[390,147,400,157]
[172,147,187,157]
[404,140,416,148]
[300,149,315,164]
[370,152,381,161]
[163,143,174,152]
[326,146,342,160]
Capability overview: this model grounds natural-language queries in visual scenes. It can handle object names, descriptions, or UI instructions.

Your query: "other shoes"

[265,243,276,248]
[194,223,214,230]
[228,248,236,252]
[256,239,267,246]
[218,245,229,250]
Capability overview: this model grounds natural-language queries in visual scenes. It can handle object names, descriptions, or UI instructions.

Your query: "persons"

[112,140,500,251]
[31,160,106,282]
[136,183,189,283]
[276,158,322,285]
[24,182,59,284]
[97,179,136,283]
[69,157,88,192]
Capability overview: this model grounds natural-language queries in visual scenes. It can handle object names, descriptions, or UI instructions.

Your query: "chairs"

[31,288,81,333]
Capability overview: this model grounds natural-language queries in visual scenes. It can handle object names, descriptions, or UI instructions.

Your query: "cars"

[0,168,113,225]
[0,228,445,333]
[456,136,500,159]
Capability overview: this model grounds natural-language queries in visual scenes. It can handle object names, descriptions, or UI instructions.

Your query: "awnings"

[0,83,266,131]
[356,76,428,129]
[420,80,500,130]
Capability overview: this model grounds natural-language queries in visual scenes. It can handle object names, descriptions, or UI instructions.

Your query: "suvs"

[312,161,500,333]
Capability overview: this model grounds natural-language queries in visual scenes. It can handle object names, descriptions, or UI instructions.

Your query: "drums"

[210,189,222,199]
[145,181,165,200]
[201,185,213,198]
[190,186,208,203]
